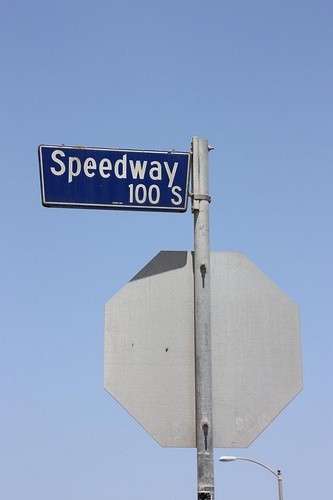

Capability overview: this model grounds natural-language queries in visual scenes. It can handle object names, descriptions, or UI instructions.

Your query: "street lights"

[219,456,284,499]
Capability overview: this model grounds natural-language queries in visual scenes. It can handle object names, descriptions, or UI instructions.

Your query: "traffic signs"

[40,147,190,211]
